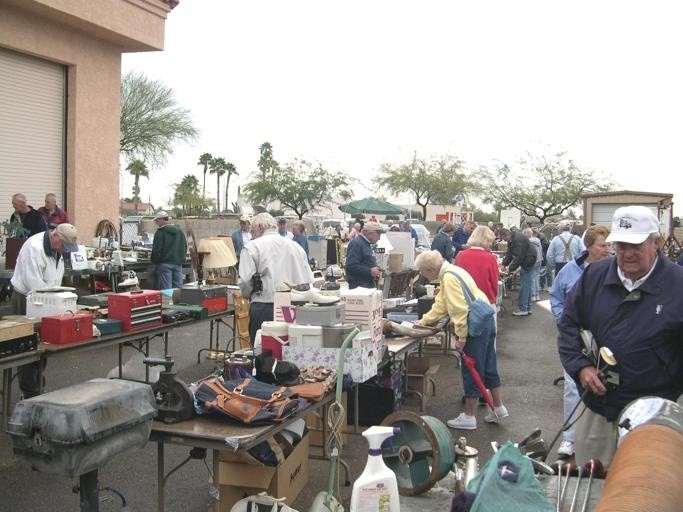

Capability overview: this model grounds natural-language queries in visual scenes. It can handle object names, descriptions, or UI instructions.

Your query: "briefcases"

[40,310,93,344]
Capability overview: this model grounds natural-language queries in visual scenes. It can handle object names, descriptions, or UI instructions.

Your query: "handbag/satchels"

[255,274,262,293]
[467,298,495,338]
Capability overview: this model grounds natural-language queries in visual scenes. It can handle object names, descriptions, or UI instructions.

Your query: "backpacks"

[195,375,301,424]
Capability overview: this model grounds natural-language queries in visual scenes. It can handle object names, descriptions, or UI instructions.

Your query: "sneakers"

[484,406,509,422]
[512,311,529,316]
[557,440,575,455]
[446,413,477,430]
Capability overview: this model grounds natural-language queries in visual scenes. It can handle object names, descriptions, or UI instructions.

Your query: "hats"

[604,205,659,244]
[364,222,383,232]
[239,216,250,224]
[152,211,168,221]
[56,223,79,252]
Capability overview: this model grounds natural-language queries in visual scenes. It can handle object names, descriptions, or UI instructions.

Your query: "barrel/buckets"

[261,321,288,360]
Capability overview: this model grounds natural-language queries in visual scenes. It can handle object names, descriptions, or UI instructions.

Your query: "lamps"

[196,236,234,285]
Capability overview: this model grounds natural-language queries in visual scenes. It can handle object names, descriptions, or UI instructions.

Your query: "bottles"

[457,445,478,486]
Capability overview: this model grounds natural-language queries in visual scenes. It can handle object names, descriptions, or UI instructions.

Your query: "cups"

[426,285,436,298]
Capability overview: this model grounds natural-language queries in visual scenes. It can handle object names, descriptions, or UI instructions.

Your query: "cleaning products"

[350,425,402,512]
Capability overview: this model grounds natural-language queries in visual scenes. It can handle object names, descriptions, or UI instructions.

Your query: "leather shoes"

[291,290,341,306]
[118,276,138,286]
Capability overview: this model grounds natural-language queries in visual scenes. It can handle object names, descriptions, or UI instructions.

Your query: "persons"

[8,193,46,236]
[149,209,187,290]
[449,224,502,405]
[36,192,69,232]
[8,223,79,401]
[409,250,508,431]
[232,213,681,349]
[549,225,626,456]
[556,206,682,470]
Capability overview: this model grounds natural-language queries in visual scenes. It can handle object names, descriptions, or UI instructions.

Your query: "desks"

[0,251,529,511]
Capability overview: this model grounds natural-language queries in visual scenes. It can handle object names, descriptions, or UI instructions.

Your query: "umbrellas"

[338,196,408,222]
[453,346,499,421]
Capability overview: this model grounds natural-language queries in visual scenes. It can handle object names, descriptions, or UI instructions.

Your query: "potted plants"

[0,218,28,269]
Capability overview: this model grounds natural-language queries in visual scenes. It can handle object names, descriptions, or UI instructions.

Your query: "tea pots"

[389,251,406,274]
